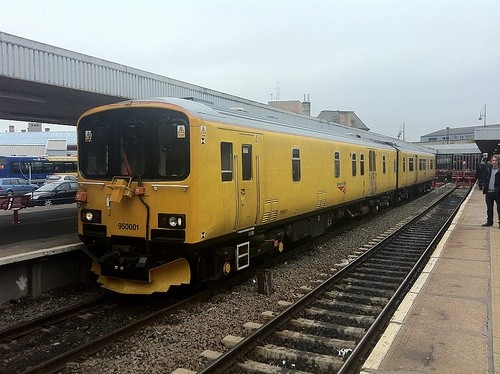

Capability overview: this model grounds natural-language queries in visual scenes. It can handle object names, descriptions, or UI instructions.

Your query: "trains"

[75,95,438,296]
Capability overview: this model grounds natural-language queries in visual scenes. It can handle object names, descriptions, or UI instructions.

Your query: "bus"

[46,154,78,177]
[0,154,55,187]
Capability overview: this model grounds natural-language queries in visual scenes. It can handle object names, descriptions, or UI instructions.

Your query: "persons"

[481,154,500,229]
[148,123,181,179]
[477,158,492,190]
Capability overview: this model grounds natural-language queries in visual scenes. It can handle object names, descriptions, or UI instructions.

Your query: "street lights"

[479,103,487,128]
[399,121,406,142]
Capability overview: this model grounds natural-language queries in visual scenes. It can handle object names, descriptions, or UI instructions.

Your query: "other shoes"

[482,224,492,227]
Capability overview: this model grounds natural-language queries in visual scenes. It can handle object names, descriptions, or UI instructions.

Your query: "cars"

[25,179,78,206]
[0,178,39,198]
[43,174,76,185]
